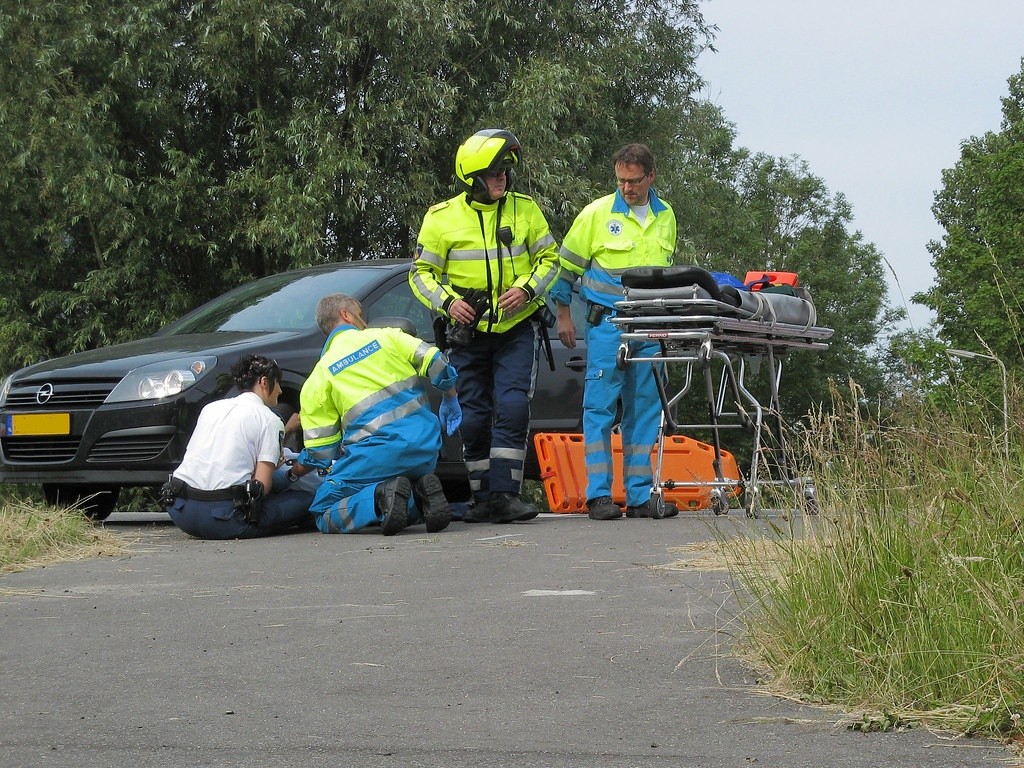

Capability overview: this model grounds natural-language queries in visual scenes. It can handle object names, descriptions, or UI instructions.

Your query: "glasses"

[614,170,649,186]
[486,167,507,178]
[257,358,278,385]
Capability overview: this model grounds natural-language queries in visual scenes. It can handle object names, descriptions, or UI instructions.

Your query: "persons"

[277,412,341,495]
[548,143,678,519]
[408,129,560,523]
[162,351,315,539]
[285,293,462,537]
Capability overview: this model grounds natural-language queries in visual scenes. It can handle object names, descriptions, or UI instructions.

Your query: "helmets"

[455,126,524,193]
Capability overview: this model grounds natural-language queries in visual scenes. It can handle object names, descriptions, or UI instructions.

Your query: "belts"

[603,307,627,319]
[169,478,246,502]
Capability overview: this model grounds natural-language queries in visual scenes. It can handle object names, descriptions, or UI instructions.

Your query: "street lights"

[943,348,1009,466]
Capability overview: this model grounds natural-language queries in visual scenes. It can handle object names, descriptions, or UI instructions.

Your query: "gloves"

[438,394,463,437]
[447,287,493,345]
[272,463,293,493]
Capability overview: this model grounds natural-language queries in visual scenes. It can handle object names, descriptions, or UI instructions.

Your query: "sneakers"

[489,492,539,525]
[625,493,679,519]
[462,498,493,524]
[376,475,411,536]
[587,496,622,520]
[413,473,452,534]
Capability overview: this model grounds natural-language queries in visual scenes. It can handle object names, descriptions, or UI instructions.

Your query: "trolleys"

[608,265,835,518]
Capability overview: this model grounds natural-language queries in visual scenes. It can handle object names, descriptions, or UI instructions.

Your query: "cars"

[0,258,673,523]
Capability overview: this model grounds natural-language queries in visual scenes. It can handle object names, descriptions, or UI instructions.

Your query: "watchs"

[288,470,299,482]
